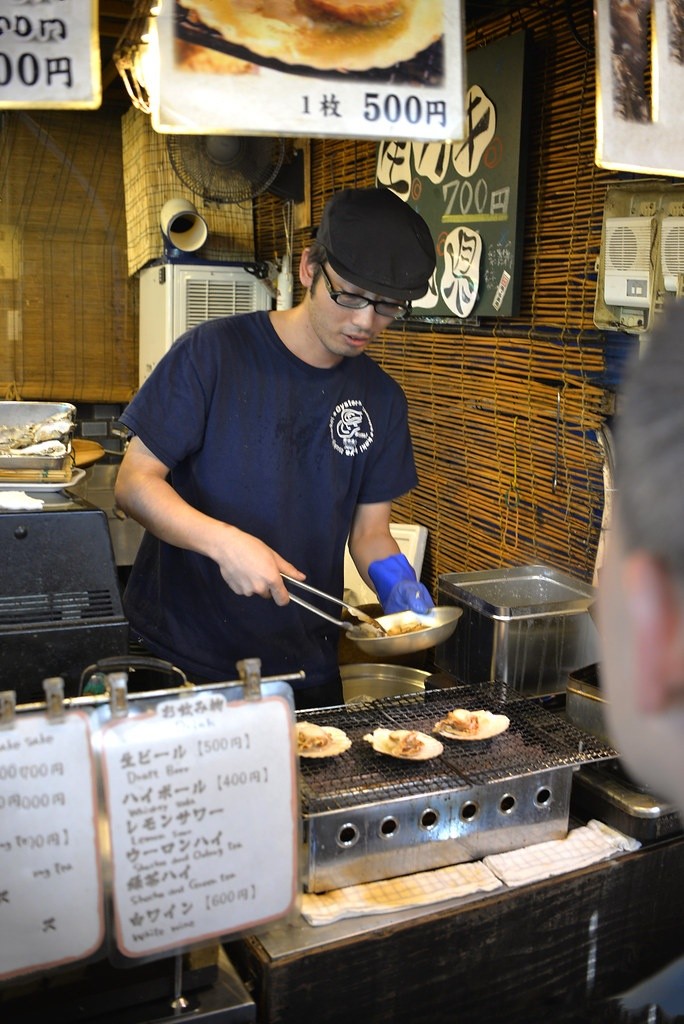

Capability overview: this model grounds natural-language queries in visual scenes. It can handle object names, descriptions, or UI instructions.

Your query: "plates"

[346,606,464,656]
[179,0,443,72]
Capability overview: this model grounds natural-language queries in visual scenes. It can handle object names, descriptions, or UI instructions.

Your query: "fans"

[165,133,313,231]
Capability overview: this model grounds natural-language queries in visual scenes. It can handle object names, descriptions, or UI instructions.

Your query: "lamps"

[113,1,154,114]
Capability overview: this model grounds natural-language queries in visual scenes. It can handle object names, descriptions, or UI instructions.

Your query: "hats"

[315,187,436,301]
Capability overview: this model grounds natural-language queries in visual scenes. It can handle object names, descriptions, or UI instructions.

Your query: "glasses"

[318,262,412,320]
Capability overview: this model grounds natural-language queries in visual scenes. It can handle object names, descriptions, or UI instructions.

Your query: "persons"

[593,290,684,811]
[114,186,437,708]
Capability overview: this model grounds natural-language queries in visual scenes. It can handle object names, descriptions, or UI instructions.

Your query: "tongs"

[279,572,388,639]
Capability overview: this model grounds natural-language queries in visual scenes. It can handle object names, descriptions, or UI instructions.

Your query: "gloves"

[368,553,435,615]
